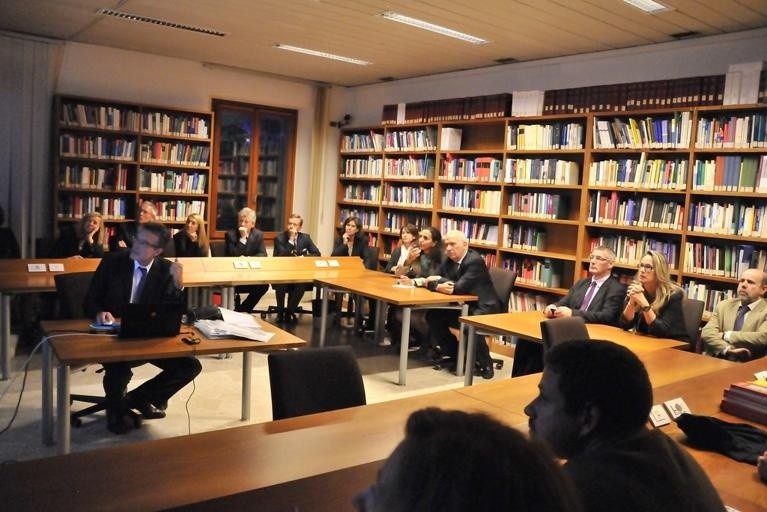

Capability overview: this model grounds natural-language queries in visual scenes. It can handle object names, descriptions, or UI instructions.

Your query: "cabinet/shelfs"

[216,131,282,230]
[48,91,217,254]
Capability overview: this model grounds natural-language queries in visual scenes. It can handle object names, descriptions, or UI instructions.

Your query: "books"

[588,111,692,285]
[502,123,584,312]
[341,127,437,258]
[718,369,766,428]
[438,128,502,270]
[192,319,233,340]
[381,60,766,125]
[681,115,766,319]
[217,116,285,222]
[57,95,212,259]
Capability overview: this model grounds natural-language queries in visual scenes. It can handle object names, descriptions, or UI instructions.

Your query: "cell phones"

[551,308,557,315]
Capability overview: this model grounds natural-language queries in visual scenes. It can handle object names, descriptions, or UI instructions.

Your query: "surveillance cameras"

[329,120,344,127]
[343,112,352,121]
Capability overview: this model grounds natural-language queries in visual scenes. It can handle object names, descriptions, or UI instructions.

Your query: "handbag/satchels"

[190,303,221,321]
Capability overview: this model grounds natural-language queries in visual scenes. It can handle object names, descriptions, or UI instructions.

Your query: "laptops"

[113,299,184,339]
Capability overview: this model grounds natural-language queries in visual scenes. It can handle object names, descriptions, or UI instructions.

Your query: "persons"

[268,214,323,323]
[392,225,443,364]
[80,221,203,436]
[511,246,628,379]
[698,268,767,361]
[329,216,373,271]
[521,338,727,512]
[35,211,112,314]
[418,229,510,379]
[229,196,244,212]
[216,193,238,230]
[222,206,271,313]
[348,406,580,512]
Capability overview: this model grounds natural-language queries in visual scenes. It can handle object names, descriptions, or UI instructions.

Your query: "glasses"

[131,232,160,251]
[588,254,612,263]
[637,264,656,272]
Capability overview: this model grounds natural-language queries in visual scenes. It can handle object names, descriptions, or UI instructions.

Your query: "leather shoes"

[481,358,494,378]
[107,404,128,434]
[276,310,285,323]
[128,394,166,419]
[287,311,297,324]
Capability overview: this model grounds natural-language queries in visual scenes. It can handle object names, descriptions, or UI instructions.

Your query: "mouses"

[102,320,113,325]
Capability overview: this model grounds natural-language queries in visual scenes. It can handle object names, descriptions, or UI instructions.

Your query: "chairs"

[209,242,267,320]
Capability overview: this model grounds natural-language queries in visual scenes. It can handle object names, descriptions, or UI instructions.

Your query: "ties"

[734,306,751,331]
[137,267,148,304]
[579,281,597,311]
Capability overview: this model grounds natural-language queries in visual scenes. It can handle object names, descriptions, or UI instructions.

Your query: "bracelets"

[172,283,185,292]
